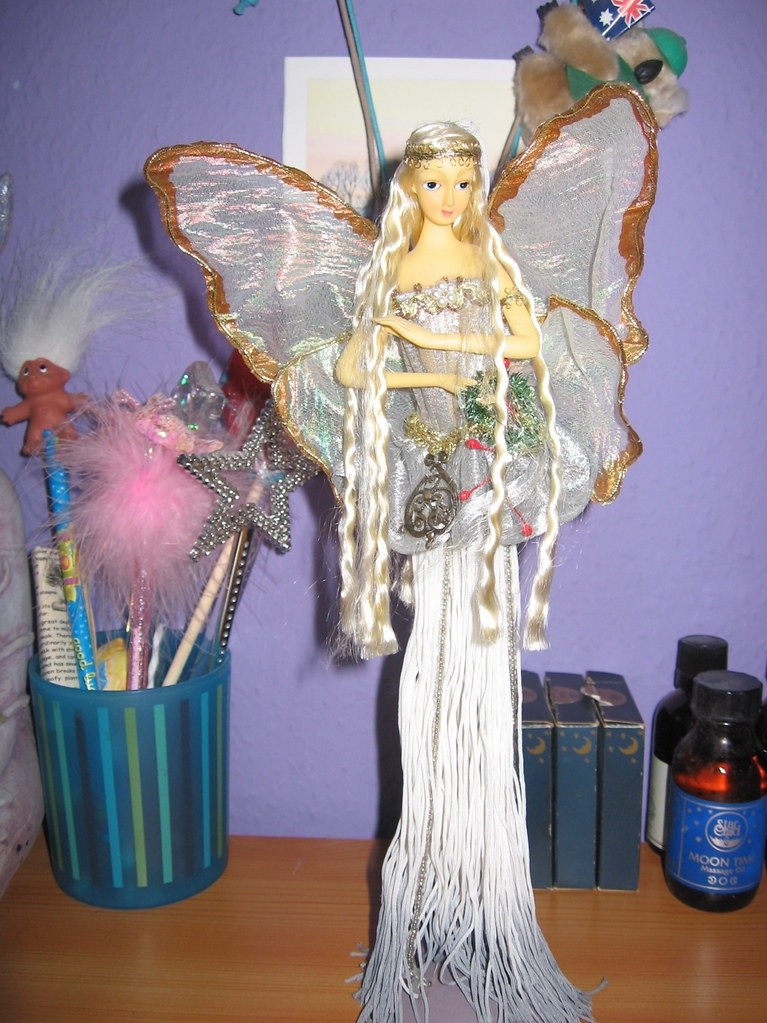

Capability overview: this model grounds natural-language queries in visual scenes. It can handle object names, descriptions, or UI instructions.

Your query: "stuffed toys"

[513,0,688,151]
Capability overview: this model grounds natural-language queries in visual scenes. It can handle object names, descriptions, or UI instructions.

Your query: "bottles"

[646,635,767,913]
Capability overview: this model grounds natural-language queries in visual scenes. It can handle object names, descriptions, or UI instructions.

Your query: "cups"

[28,629,231,909]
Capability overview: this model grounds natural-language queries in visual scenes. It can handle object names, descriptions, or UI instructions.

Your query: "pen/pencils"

[42,428,278,692]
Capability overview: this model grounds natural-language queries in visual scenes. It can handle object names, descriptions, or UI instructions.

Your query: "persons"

[331,123,591,1023]
[0,358,92,455]
